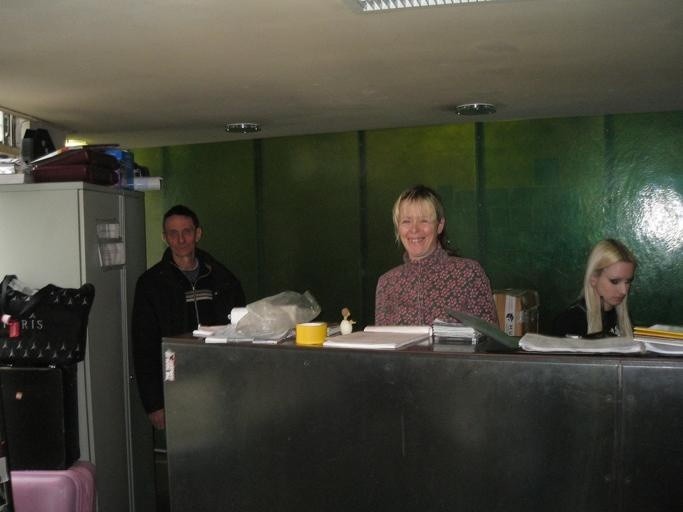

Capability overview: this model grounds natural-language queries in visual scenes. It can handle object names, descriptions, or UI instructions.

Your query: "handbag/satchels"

[0,275,95,366]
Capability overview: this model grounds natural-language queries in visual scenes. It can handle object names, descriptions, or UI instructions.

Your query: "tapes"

[295,321,328,345]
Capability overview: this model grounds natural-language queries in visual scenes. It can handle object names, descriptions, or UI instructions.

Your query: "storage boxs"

[492,288,539,337]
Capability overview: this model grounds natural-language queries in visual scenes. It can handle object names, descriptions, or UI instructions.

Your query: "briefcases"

[32,149,119,185]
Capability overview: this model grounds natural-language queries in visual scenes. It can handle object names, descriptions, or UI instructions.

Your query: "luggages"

[0,367,99,511]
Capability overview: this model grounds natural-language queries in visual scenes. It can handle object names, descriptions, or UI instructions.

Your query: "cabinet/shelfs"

[0,182,157,512]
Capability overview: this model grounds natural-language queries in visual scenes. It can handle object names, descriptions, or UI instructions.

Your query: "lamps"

[224,122,261,133]
[456,103,496,116]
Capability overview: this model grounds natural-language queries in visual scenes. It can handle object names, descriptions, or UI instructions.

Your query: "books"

[431,318,484,339]
[30,142,119,164]
[190,322,232,337]
[633,325,682,341]
[517,332,682,357]
[322,323,432,350]
[0,173,38,185]
[203,336,252,345]
[0,161,32,174]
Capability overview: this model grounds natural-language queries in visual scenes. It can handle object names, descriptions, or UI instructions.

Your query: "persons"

[551,238,635,339]
[373,182,501,333]
[130,201,248,436]
